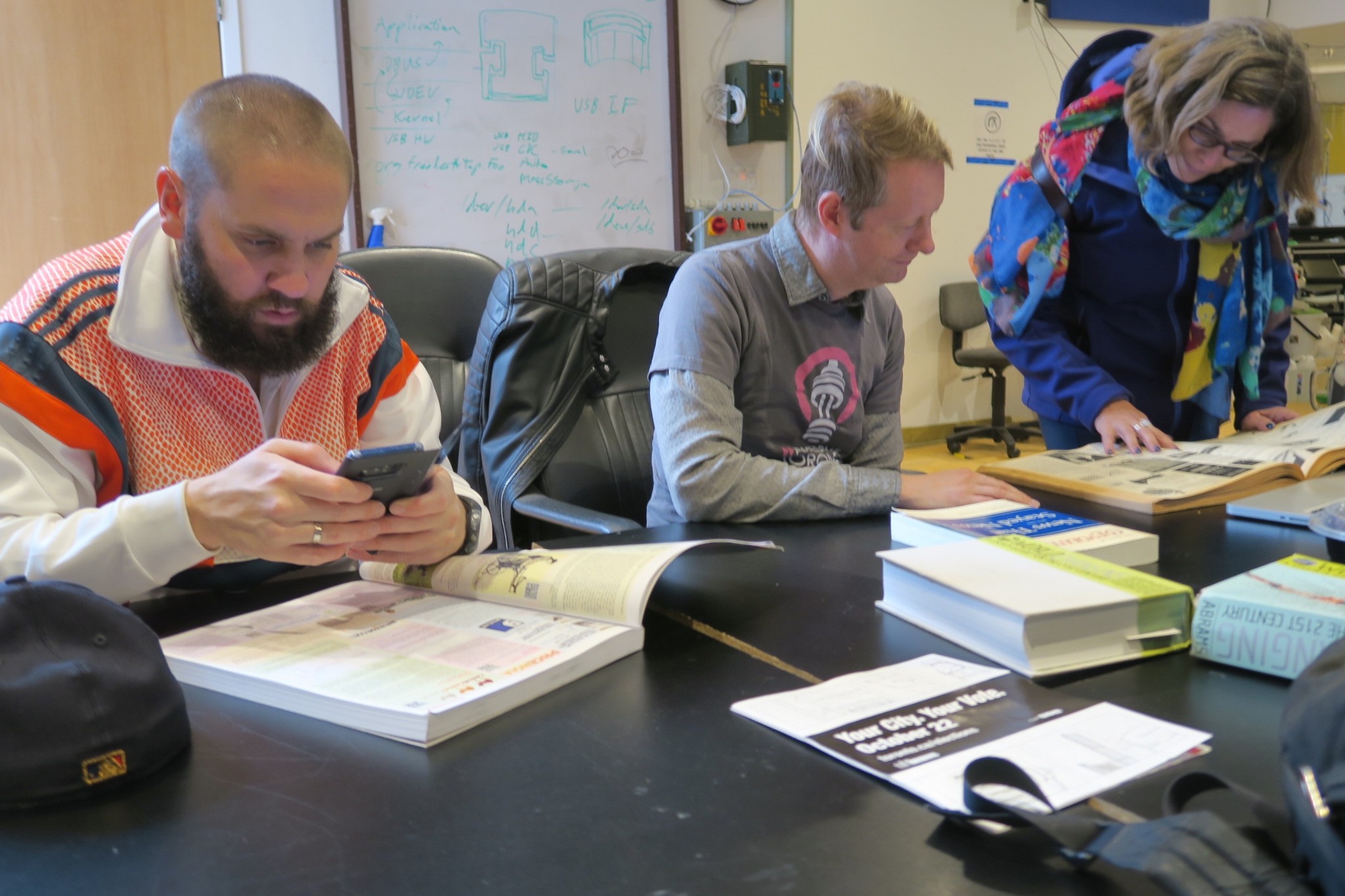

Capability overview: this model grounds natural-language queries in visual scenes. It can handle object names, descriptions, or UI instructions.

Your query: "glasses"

[1188,124,1271,165]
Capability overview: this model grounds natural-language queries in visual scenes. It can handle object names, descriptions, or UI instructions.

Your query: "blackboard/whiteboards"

[333,0,685,269]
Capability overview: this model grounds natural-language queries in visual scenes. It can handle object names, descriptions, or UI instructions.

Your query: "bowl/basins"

[1308,500,1345,565]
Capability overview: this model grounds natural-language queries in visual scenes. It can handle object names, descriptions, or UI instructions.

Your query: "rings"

[1132,417,1152,432]
[313,522,323,546]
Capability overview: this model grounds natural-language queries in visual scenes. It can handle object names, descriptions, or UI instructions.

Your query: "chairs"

[340,246,502,473]
[939,281,1030,459]
[460,247,694,551]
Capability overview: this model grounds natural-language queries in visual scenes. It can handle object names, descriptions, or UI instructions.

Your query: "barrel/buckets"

[1283,313,1328,361]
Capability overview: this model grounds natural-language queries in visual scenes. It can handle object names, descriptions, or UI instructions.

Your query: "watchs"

[456,493,483,555]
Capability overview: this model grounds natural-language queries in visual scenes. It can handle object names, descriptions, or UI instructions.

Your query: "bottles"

[1285,356,1316,402]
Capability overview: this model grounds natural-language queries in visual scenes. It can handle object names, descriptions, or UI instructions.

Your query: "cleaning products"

[366,206,395,249]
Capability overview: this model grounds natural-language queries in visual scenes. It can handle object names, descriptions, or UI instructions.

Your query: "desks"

[0,488,1345,896]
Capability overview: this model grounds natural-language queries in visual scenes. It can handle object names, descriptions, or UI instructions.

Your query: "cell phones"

[336,441,442,517]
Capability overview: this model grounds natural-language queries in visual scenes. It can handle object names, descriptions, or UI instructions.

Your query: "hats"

[0,576,192,815]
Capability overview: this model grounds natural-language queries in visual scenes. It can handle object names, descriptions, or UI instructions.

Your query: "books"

[152,403,1345,751]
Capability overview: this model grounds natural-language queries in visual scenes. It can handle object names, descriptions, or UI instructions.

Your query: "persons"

[0,75,493,604]
[645,78,1040,527]
[970,16,1322,456]
